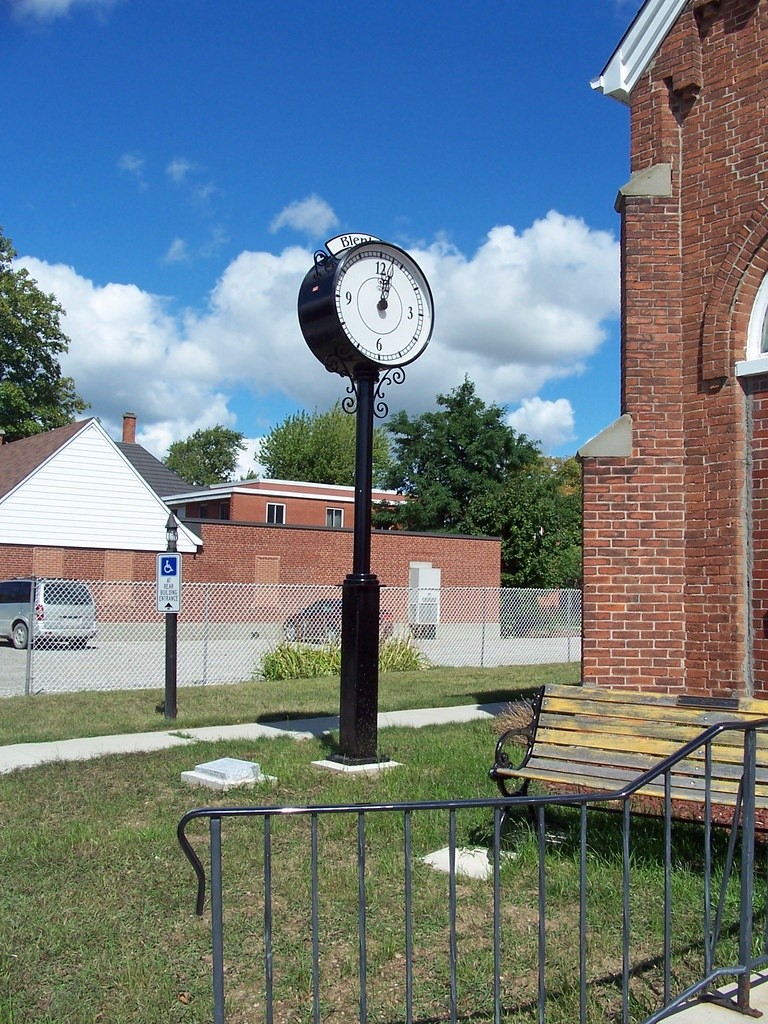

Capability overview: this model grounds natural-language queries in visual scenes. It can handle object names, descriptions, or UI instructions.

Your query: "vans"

[0,577,98,649]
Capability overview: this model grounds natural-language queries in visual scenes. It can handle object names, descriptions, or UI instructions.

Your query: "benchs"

[472,682,768,848]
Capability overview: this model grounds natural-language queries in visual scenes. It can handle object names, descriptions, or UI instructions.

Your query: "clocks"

[297,234,438,378]
